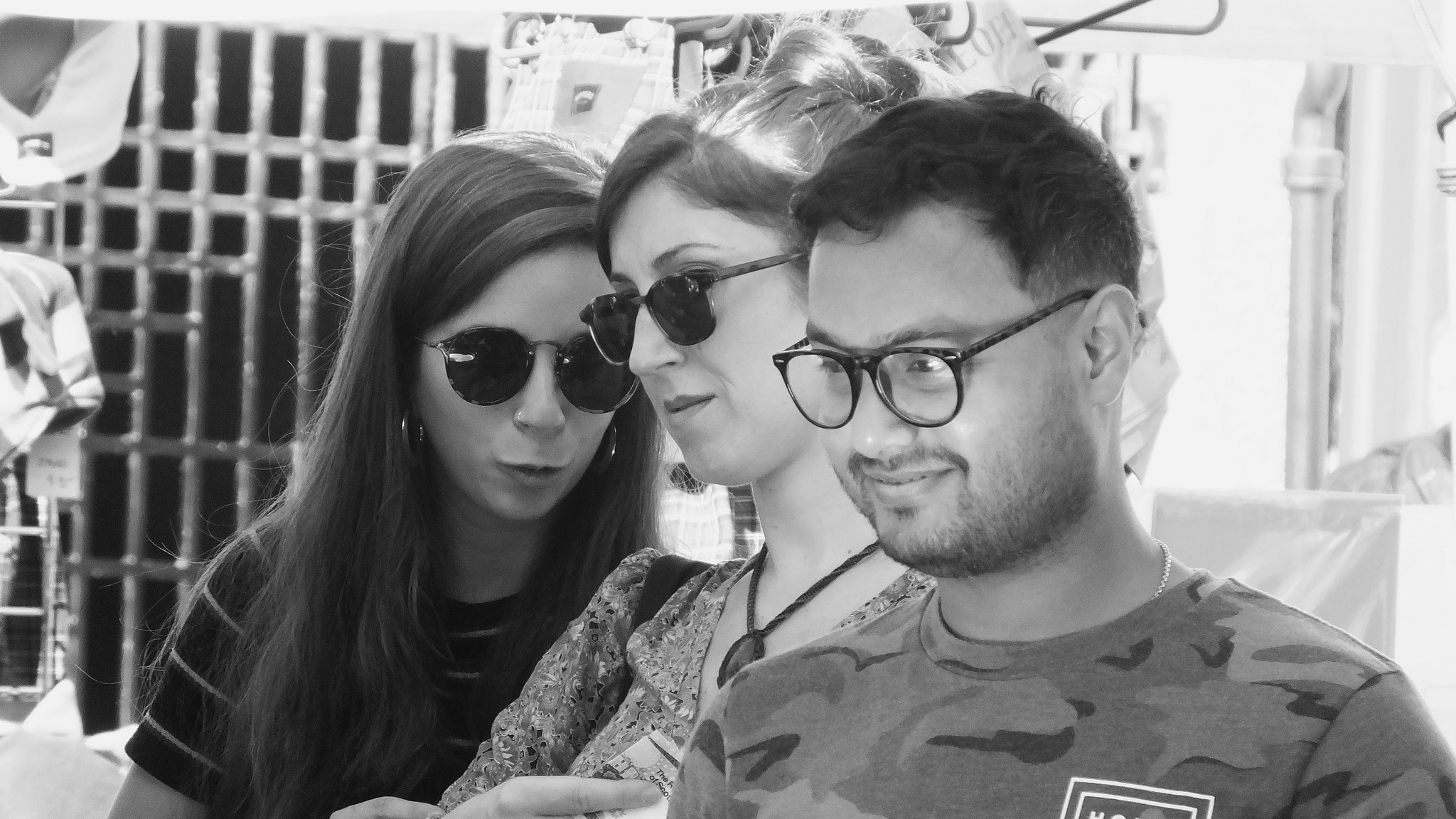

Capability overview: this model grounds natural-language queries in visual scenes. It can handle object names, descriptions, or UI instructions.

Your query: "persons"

[108,127,689,819]
[665,91,1456,818]
[436,19,924,818]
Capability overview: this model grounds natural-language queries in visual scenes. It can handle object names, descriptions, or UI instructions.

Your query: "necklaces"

[1158,542,1174,604]
[718,537,882,690]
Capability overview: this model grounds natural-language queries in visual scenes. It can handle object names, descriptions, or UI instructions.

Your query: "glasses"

[772,291,1099,429]
[402,326,638,413]
[579,245,811,367]
